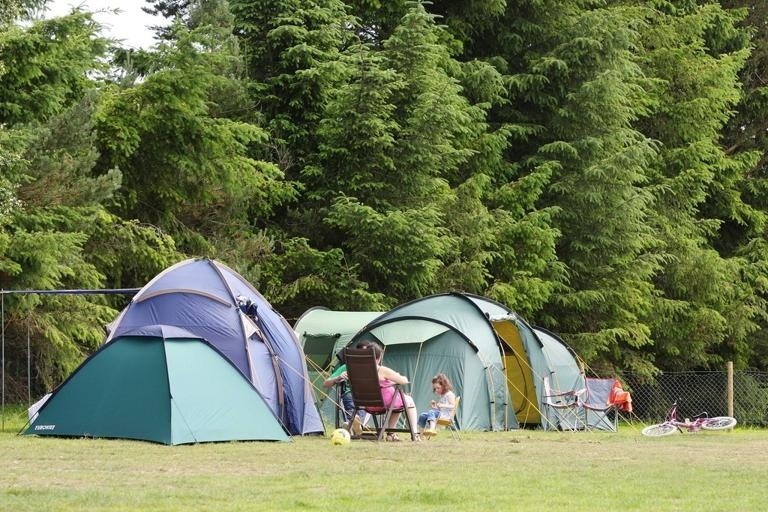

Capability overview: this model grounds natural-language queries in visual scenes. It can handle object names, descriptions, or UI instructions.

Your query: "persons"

[323,341,369,437]
[366,342,422,442]
[417,372,456,437]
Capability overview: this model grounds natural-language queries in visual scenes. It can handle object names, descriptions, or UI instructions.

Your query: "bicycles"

[639,396,740,438]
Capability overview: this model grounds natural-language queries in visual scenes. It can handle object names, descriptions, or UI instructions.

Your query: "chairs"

[335,346,461,444]
[539,376,620,432]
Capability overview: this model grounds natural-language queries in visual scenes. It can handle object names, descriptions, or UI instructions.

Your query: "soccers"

[331,429,350,445]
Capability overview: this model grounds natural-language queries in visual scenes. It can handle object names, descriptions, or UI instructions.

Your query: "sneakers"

[411,429,438,441]
[386,433,400,441]
[342,422,354,436]
[353,415,363,436]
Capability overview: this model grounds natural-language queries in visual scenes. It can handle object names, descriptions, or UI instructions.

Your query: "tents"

[289,291,614,433]
[15,322,295,448]
[105,256,325,435]
